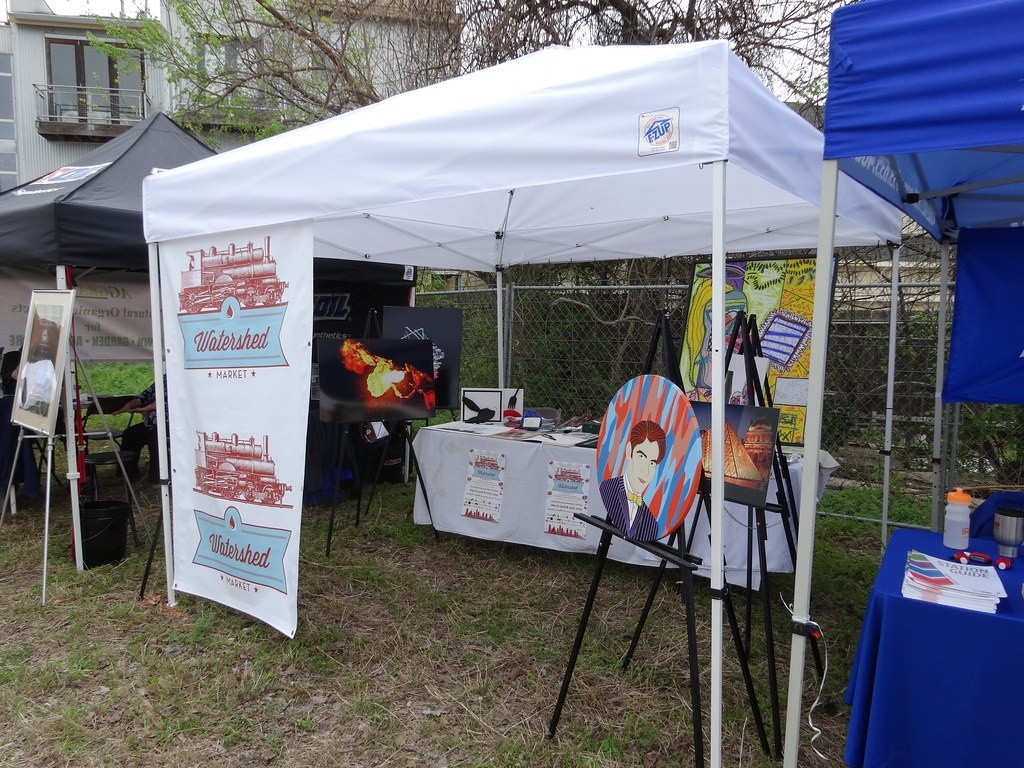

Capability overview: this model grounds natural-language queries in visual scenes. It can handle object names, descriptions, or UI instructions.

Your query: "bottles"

[944,488,973,550]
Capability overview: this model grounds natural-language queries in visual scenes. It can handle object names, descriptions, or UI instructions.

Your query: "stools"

[86,452,141,547]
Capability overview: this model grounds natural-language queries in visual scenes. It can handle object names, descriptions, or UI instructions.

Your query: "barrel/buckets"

[77,502,128,566]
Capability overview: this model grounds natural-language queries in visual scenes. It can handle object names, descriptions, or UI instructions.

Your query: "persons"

[1,344,23,488]
[111,373,169,489]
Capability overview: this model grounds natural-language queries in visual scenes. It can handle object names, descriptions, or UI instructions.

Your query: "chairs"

[65,397,144,497]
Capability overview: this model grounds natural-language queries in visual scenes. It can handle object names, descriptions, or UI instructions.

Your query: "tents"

[0,114,221,576]
[140,39,905,768]
[784,0,1023,768]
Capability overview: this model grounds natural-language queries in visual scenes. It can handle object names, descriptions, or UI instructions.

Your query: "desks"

[410,419,841,590]
[842,528,1024,768]
